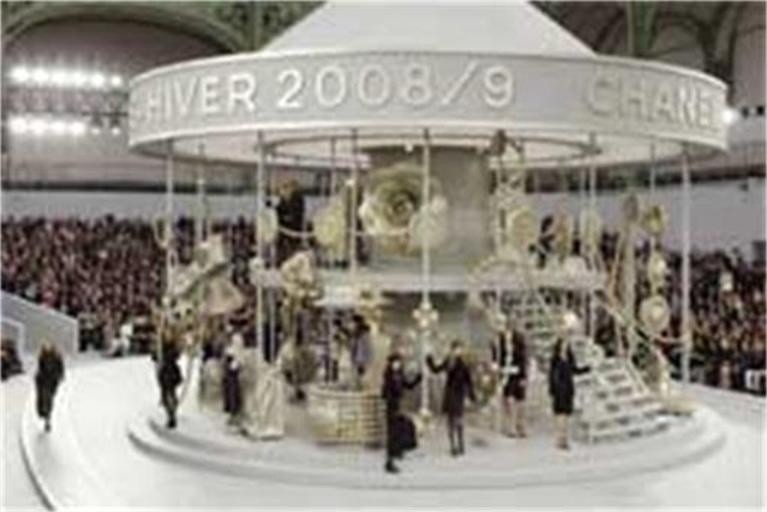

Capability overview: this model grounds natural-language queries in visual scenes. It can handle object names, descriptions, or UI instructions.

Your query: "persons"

[533,217,767,396]
[482,309,531,439]
[267,179,303,264]
[32,335,64,431]
[1,214,314,358]
[375,350,425,476]
[2,338,24,381]
[545,326,591,452]
[149,334,188,430]
[420,335,477,459]
[218,330,251,428]
[321,314,375,383]
